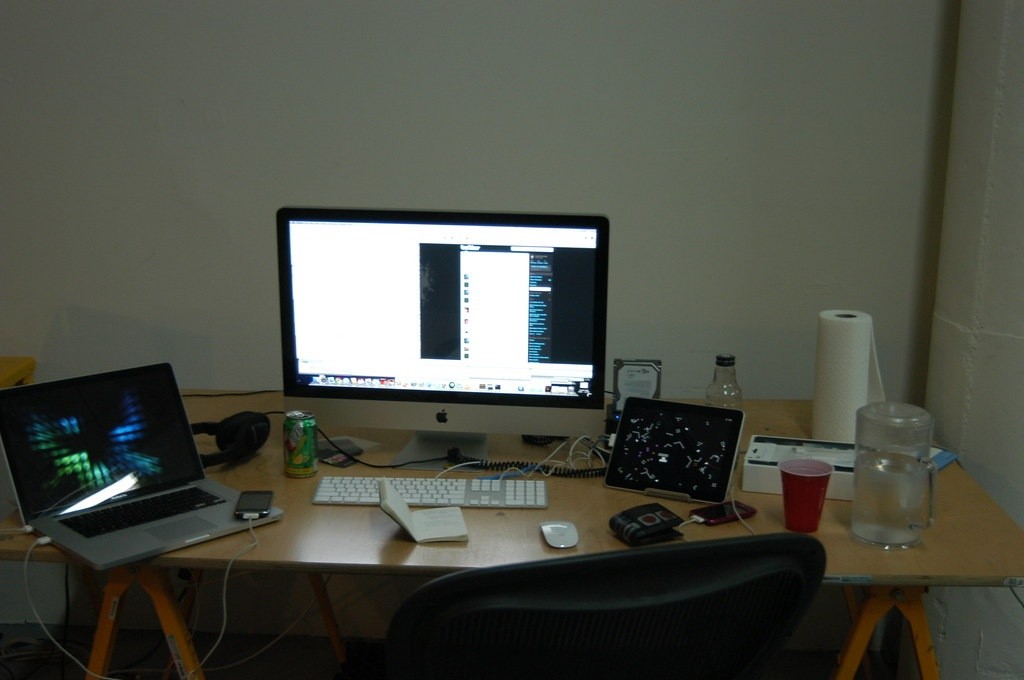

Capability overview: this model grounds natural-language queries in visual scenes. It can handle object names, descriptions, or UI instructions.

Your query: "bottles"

[705,354,743,409]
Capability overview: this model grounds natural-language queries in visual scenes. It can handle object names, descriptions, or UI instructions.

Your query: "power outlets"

[175,567,202,587]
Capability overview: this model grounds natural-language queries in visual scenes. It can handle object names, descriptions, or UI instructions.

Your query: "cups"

[852,401,935,548]
[778,458,834,533]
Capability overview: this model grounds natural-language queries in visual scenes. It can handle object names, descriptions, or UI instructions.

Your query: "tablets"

[604,397,746,503]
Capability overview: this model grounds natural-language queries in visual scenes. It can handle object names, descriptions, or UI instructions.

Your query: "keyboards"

[313,476,548,510]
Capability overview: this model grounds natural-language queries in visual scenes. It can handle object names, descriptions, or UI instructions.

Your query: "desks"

[0,389,1024,680]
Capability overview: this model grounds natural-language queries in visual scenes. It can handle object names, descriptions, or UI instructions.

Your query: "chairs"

[385,534,827,680]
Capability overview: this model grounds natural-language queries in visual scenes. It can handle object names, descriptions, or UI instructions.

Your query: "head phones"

[191,412,271,469]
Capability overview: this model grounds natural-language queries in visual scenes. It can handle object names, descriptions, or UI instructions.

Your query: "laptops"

[0,361,282,572]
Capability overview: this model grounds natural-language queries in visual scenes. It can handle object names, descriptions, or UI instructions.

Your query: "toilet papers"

[812,308,886,445]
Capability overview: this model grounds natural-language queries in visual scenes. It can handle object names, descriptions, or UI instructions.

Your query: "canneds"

[283,409,320,478]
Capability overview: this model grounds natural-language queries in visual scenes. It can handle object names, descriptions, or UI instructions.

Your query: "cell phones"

[234,490,274,518]
[689,501,758,525]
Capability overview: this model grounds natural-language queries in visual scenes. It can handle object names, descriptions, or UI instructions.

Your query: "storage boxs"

[742,435,880,501]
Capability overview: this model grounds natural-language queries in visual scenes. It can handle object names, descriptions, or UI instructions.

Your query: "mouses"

[540,521,579,549]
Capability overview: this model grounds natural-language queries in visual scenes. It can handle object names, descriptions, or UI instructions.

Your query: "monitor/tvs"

[274,207,608,471]
